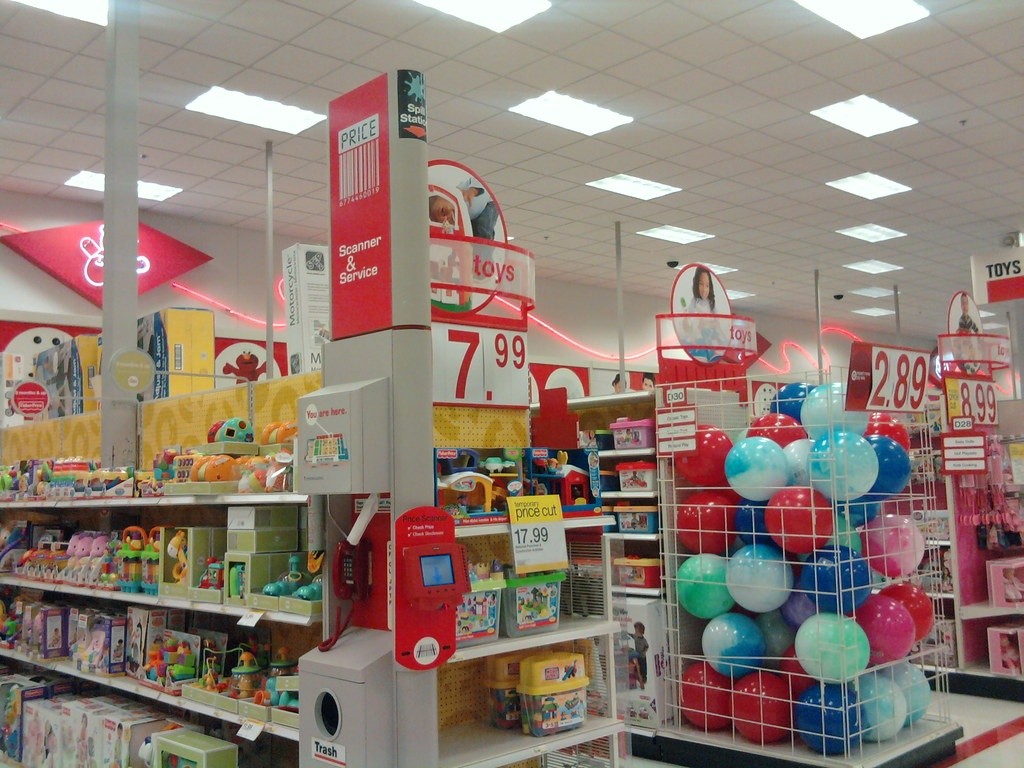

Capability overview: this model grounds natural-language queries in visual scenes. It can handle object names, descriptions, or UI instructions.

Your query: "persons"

[612,374,627,395]
[626,621,649,684]
[641,372,655,392]
[957,292,980,347]
[682,267,728,360]
[430,177,499,280]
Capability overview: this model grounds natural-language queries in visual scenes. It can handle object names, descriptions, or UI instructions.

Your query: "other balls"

[675,379,938,753]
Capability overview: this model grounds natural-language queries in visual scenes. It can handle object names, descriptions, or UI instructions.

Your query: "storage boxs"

[157,524,211,598]
[225,530,299,555]
[104,713,174,768]
[983,554,1024,608]
[168,609,187,636]
[592,427,613,449]
[188,585,224,605]
[101,618,126,673]
[248,592,279,612]
[157,728,240,768]
[519,651,592,734]
[611,505,657,530]
[279,594,327,614]
[224,553,270,606]
[500,564,571,636]
[228,506,300,529]
[986,620,1024,681]
[192,440,259,457]
[23,698,63,768]
[211,524,224,562]
[269,551,289,583]
[598,504,611,532]
[21,604,40,652]
[615,555,662,586]
[125,606,166,679]
[446,578,506,648]
[60,695,153,768]
[608,420,653,446]
[613,460,657,491]
[161,481,240,496]
[0,674,47,765]
[598,470,616,489]
[24,669,76,699]
[35,607,67,659]
[481,656,520,728]
[587,595,671,726]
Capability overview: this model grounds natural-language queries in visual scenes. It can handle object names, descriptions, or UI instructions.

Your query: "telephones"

[332,539,373,603]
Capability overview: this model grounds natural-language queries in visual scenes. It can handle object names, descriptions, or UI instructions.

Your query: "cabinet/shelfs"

[0,440,1024,768]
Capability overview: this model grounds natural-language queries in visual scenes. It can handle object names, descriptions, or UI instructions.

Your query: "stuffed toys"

[65,531,108,576]
